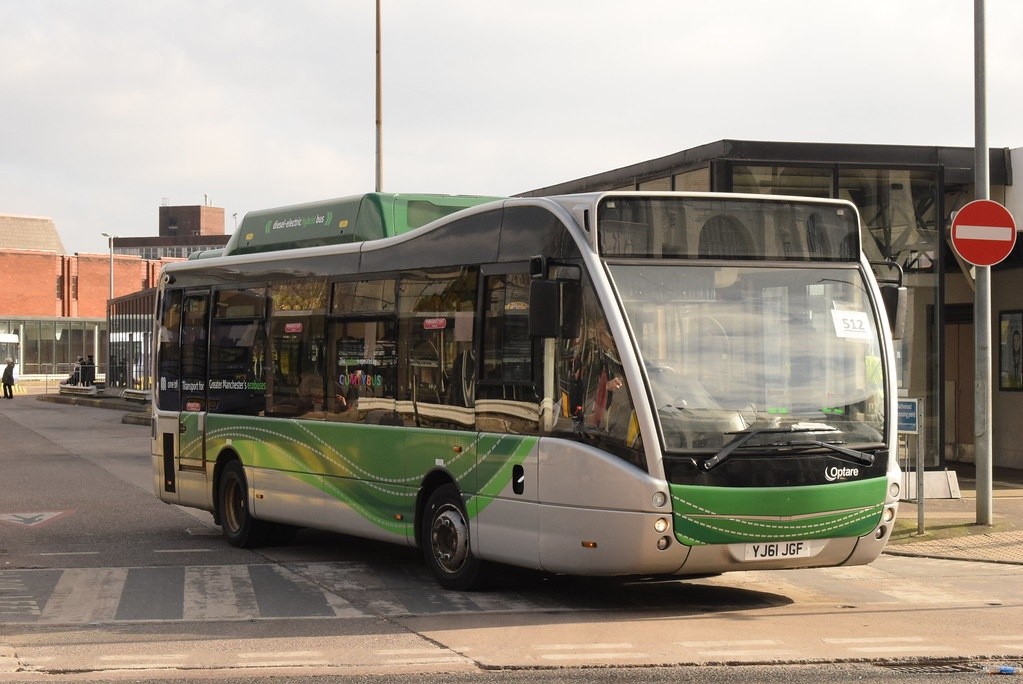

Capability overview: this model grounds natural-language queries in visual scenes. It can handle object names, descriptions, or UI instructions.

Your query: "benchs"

[474,380,541,405]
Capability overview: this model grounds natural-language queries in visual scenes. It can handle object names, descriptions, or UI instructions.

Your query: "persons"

[580,313,663,430]
[2,357,14,399]
[204,340,513,431]
[61,354,135,387]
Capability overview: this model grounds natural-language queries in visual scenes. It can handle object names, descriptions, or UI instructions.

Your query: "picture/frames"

[998,309,1023,391]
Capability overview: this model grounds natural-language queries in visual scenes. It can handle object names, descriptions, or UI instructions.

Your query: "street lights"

[102,232,117,299]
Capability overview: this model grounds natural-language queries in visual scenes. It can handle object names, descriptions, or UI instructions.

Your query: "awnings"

[0,333,20,346]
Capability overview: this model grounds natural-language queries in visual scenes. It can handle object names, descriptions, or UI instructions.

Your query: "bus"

[151,190,911,592]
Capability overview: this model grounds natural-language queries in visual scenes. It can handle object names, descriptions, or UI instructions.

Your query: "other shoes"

[3,395,8,398]
[7,396,13,399]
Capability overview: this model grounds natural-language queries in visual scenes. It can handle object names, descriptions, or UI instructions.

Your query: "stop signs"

[950,197,1019,265]
